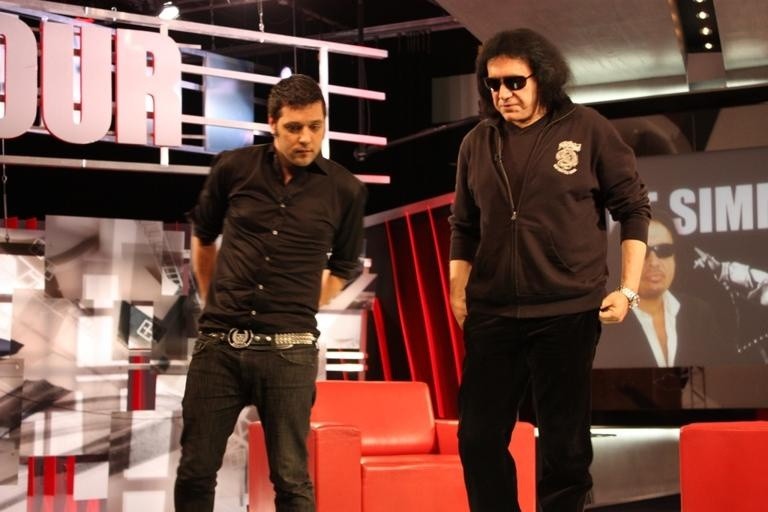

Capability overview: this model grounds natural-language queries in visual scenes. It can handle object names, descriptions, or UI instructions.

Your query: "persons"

[447,26,652,511]
[174,74,371,512]
[594,206,730,373]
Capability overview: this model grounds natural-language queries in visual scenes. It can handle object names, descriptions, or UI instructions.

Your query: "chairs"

[677,417,768,512]
[244,378,539,512]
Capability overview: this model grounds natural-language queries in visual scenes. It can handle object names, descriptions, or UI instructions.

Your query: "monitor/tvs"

[576,84,768,425]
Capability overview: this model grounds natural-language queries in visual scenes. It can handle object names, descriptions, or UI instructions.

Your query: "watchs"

[617,287,640,310]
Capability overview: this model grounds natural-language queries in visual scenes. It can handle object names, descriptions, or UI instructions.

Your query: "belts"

[200,327,317,350]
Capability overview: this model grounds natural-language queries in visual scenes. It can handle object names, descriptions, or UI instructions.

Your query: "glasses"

[482,73,535,91]
[645,243,675,260]
